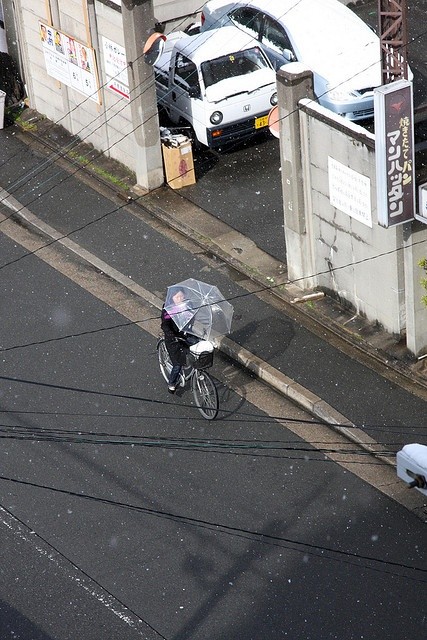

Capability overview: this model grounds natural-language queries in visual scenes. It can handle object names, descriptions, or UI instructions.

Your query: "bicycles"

[156,322,226,422]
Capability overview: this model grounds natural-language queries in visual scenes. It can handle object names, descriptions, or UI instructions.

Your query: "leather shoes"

[167,386,176,395]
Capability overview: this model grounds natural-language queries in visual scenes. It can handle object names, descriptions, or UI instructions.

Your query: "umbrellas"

[163,278,235,342]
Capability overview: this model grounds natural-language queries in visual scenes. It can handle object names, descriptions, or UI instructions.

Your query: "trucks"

[154,25,289,151]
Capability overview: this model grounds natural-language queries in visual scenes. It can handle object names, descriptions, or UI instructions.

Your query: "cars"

[196,0,415,123]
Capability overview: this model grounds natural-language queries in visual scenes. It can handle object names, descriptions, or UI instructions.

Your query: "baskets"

[188,347,215,370]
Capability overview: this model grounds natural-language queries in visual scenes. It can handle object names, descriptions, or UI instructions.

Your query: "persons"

[161,287,198,391]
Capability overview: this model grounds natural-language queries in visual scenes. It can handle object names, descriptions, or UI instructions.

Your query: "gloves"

[172,335,189,350]
